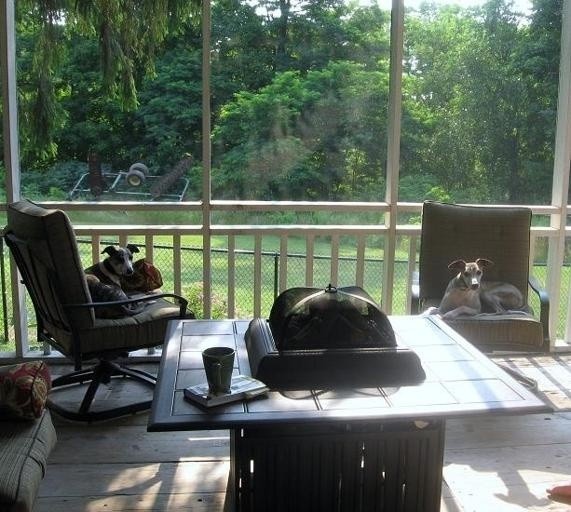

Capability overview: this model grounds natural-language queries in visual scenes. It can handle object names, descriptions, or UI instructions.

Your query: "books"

[184,375,272,410]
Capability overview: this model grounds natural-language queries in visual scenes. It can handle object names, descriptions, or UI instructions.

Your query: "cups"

[203,348,234,394]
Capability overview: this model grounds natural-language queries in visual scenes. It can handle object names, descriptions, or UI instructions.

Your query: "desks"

[145,311,556,512]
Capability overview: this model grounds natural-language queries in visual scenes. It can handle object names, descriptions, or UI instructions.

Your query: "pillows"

[0,358,54,424]
[123,258,163,294]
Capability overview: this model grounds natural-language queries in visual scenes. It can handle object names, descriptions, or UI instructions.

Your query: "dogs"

[83,244,156,320]
[423,258,528,320]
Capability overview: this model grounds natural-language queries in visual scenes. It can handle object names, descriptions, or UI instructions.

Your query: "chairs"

[0,197,195,424]
[404,200,553,360]
